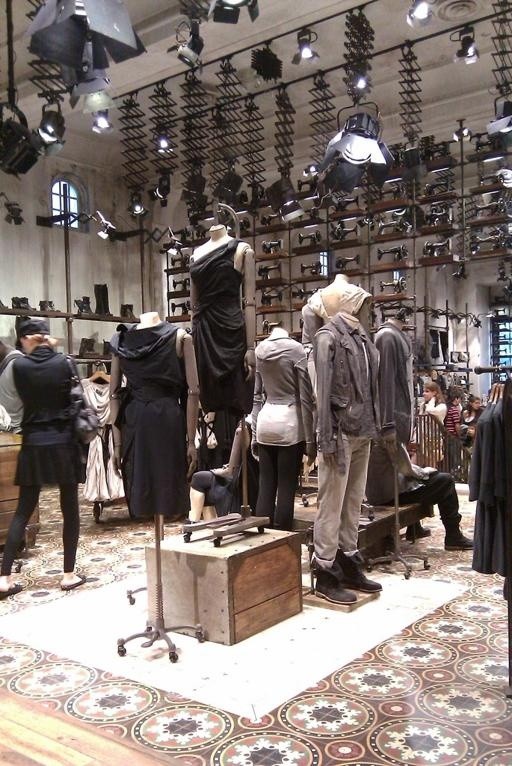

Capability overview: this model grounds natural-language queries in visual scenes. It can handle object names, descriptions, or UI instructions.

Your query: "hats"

[16,320,50,337]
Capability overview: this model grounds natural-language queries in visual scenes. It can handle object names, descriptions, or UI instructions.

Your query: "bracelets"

[113,442,123,447]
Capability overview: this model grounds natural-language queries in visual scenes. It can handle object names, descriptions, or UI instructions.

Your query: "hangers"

[78,359,112,386]
[480,365,506,420]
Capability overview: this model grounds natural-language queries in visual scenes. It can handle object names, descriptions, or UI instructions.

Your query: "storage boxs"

[145,527,302,646]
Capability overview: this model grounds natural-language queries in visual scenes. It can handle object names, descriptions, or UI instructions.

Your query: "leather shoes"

[1,584,22,600]
[61,575,86,590]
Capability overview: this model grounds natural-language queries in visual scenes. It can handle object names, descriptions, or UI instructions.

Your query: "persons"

[105,311,194,521]
[184,222,258,416]
[0,338,29,435]
[371,317,413,455]
[362,426,474,553]
[187,416,259,524]
[314,313,383,606]
[255,273,373,532]
[420,381,485,484]
[0,319,92,600]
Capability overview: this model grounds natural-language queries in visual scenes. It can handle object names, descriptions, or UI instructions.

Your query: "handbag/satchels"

[75,408,99,444]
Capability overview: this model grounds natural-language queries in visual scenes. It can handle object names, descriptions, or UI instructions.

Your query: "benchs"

[293,497,434,555]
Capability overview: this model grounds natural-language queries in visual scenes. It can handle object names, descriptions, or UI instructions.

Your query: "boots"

[11,297,35,311]
[40,301,59,311]
[94,284,110,316]
[75,296,94,316]
[406,519,431,540]
[315,571,357,605]
[442,522,475,550]
[103,341,111,355]
[340,556,382,592]
[79,337,99,356]
[121,303,134,316]
